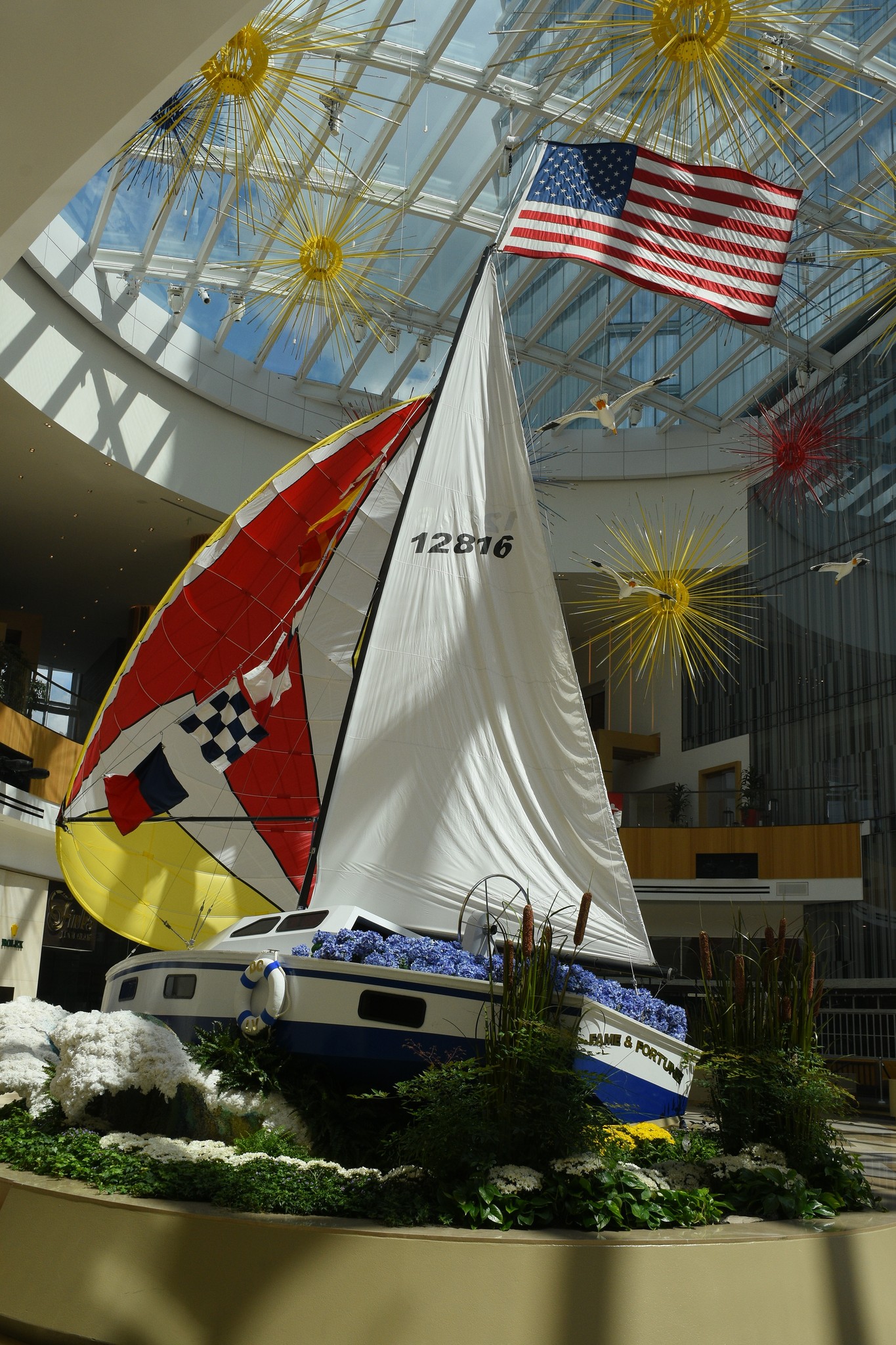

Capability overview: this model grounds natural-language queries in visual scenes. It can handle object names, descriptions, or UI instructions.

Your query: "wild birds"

[810,553,871,583]
[533,374,680,436]
[589,558,678,602]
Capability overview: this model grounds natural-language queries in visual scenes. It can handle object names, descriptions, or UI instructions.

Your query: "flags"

[495,139,804,327]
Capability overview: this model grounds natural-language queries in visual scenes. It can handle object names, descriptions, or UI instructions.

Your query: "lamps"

[351,316,369,343]
[167,283,185,315]
[198,287,211,304]
[857,75,863,127]
[510,356,522,367]
[416,334,433,363]
[608,394,618,401]
[758,33,780,71]
[779,355,812,388]
[228,290,245,322]
[424,81,430,132]
[126,280,141,299]
[784,55,798,72]
[352,210,356,247]
[628,402,644,426]
[385,323,402,353]
[293,331,296,344]
[183,178,189,216]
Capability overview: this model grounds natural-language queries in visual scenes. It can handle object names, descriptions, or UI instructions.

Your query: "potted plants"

[737,765,765,826]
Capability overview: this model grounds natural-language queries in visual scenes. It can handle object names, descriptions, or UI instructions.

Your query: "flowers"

[291,930,687,1042]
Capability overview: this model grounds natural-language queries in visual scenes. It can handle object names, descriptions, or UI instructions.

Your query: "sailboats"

[52,131,807,1136]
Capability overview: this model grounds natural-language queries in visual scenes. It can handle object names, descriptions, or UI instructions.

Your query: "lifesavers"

[234,956,286,1036]
